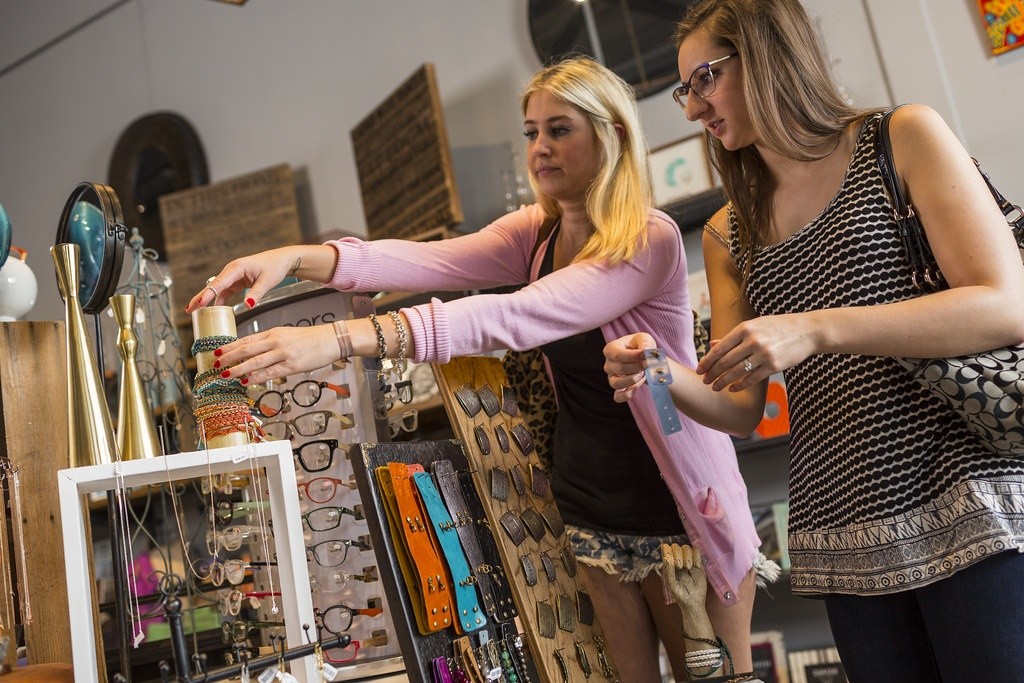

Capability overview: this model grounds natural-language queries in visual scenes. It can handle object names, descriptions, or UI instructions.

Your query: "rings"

[206,276,218,299]
[744,358,753,374]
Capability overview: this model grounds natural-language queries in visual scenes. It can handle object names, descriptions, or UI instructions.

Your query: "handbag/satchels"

[500,215,561,484]
[873,103,1024,454]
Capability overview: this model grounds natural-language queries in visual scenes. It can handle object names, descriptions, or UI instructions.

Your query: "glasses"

[190,318,388,680]
[672,53,738,109]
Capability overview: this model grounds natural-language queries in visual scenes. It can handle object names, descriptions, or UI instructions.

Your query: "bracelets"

[332,320,354,364]
[387,310,407,381]
[680,623,735,677]
[367,313,387,380]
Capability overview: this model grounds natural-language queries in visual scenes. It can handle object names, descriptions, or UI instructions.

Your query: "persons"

[602,1,1024,683]
[183,52,762,683]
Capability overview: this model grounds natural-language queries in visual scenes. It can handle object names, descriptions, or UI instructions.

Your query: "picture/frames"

[644,131,717,210]
